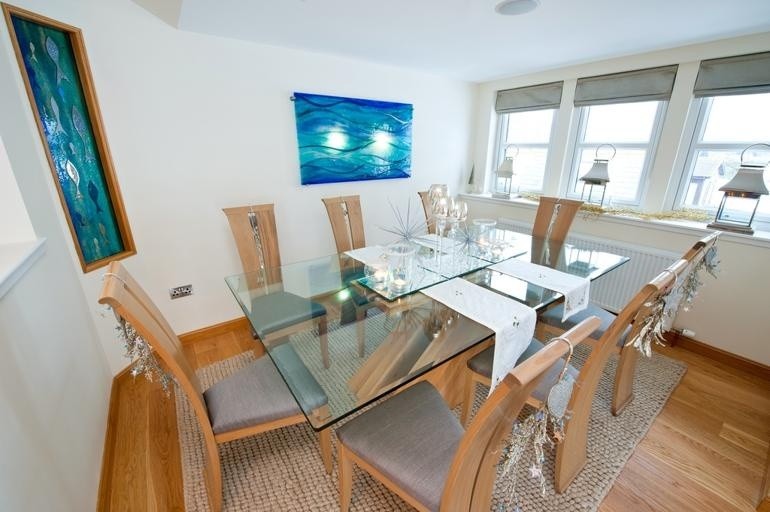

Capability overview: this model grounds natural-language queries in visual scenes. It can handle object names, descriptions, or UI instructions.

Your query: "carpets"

[169,309,690,511]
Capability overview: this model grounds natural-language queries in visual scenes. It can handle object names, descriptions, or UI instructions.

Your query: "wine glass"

[425,184,467,270]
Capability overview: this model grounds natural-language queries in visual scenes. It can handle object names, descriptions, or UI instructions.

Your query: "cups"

[363,245,415,293]
[469,216,506,262]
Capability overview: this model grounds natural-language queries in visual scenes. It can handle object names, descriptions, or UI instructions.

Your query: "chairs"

[336,314,605,512]
[461,258,692,494]
[537,229,724,418]
[532,195,585,243]
[321,193,375,358]
[223,202,331,370]
[99,258,338,512]
[418,191,445,234]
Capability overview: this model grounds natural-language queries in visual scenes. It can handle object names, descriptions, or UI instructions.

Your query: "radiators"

[497,216,696,333]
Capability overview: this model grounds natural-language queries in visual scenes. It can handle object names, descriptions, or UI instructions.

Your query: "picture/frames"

[1,1,138,275]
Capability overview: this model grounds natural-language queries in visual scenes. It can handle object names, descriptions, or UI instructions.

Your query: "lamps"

[492,143,521,200]
[706,141,770,237]
[579,143,617,210]
